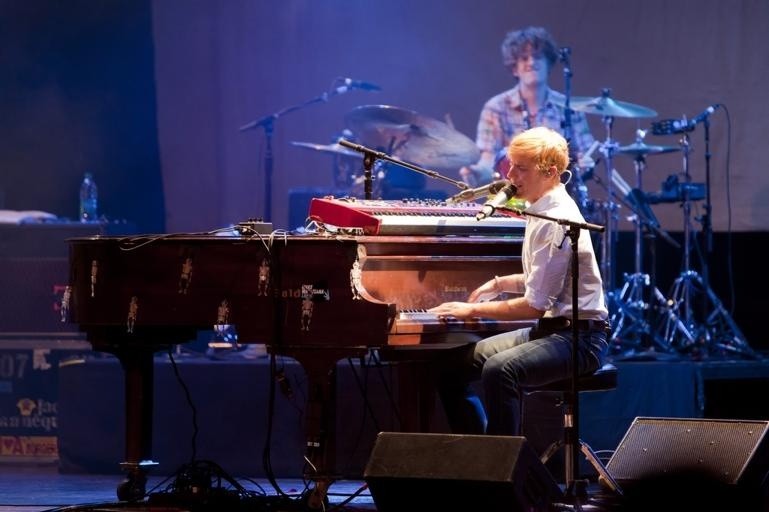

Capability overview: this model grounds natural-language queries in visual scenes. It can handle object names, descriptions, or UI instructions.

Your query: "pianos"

[309,194,529,236]
[64,235,539,512]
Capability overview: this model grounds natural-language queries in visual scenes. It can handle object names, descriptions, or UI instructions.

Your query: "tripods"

[595,121,753,357]
[538,232,624,501]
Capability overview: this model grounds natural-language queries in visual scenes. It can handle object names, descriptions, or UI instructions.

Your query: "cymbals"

[549,95,658,119]
[615,142,680,154]
[291,141,366,160]
[345,105,481,168]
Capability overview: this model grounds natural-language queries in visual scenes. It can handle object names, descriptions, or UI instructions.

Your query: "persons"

[455,23,601,206]
[421,123,610,512]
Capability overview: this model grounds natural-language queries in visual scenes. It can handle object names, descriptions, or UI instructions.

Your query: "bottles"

[79,172,98,224]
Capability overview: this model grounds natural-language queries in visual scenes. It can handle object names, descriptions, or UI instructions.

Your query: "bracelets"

[494,275,505,296]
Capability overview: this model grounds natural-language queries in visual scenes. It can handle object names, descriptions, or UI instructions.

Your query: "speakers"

[600,416,769,511]
[363,431,562,511]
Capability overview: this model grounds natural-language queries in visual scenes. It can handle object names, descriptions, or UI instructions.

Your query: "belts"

[538,317,605,332]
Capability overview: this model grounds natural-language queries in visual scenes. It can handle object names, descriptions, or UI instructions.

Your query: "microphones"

[347,79,382,92]
[685,103,718,131]
[476,184,516,220]
[450,180,508,203]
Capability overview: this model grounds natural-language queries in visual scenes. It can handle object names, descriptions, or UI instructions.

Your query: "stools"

[522,361,627,511]
